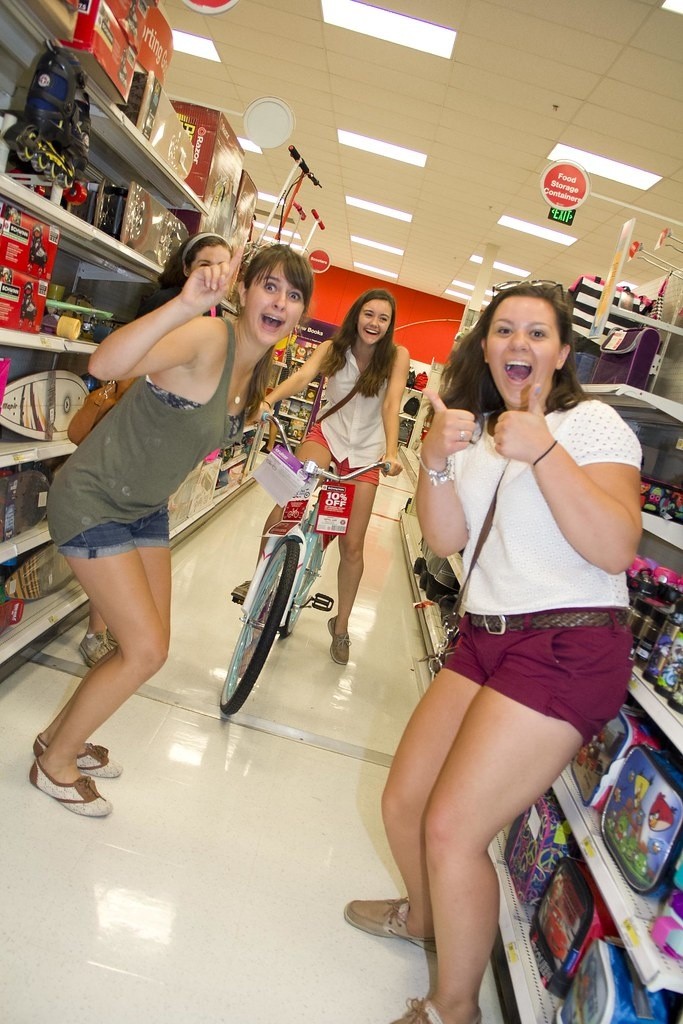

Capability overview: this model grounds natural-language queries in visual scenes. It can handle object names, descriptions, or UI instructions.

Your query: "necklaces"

[235,357,251,404]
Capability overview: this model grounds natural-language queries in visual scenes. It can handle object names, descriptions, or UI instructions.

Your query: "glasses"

[492,281,565,302]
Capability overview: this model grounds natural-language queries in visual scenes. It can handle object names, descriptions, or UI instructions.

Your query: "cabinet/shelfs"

[172,298,289,568]
[263,317,341,449]
[0,0,211,682]
[487,219,683,1023]
[398,219,499,676]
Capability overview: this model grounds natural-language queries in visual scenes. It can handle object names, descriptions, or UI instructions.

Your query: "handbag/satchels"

[590,325,661,391]
[68,378,136,446]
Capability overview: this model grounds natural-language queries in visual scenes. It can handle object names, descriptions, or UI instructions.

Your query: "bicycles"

[220,410,391,716]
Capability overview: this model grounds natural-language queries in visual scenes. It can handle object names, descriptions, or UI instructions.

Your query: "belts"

[471,611,629,635]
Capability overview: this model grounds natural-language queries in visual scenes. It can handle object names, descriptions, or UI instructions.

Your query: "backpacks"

[414,372,428,391]
[406,367,416,387]
[404,395,419,416]
[398,419,415,442]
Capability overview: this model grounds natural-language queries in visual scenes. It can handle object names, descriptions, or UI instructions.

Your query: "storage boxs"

[0,0,259,338]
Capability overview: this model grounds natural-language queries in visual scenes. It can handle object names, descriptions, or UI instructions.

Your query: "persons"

[231,290,410,665]
[78,233,232,668]
[345,285,643,1023]
[31,246,314,816]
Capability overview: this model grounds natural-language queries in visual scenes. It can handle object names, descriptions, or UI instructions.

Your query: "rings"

[461,431,465,441]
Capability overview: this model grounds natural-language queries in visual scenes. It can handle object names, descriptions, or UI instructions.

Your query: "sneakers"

[342,895,437,952]
[79,627,118,668]
[389,996,482,1024]
[231,581,251,600]
[328,615,352,665]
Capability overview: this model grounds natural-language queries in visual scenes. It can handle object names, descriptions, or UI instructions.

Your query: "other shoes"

[29,758,113,816]
[33,732,123,777]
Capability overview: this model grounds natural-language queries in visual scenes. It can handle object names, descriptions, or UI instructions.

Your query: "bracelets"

[264,400,272,409]
[417,453,452,486]
[533,440,558,465]
